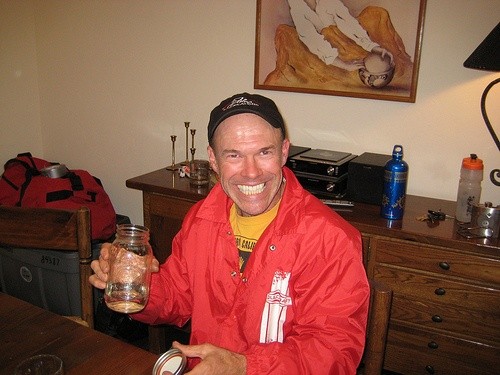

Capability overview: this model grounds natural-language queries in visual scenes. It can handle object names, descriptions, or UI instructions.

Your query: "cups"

[190,160,210,185]
[14,354,64,375]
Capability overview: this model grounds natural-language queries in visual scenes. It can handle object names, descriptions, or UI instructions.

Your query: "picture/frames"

[254,0,427,103]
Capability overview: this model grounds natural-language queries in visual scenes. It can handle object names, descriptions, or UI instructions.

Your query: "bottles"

[471,202,500,239]
[380,145,408,219]
[455,153,483,223]
[103,224,152,313]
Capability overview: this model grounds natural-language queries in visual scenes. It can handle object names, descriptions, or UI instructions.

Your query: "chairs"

[0,205,93,327]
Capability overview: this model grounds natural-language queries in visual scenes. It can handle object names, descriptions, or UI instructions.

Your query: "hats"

[207,93,285,141]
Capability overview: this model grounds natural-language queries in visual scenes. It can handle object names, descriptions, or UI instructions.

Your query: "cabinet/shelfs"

[124,162,500,375]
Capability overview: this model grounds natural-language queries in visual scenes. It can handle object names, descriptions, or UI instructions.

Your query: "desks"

[0,289,159,375]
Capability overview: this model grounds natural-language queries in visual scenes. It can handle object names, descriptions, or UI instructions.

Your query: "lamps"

[463,19,500,185]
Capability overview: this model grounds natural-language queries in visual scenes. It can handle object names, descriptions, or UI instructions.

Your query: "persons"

[89,92,370,375]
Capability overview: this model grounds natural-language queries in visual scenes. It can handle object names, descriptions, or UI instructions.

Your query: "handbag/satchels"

[0,152,118,242]
